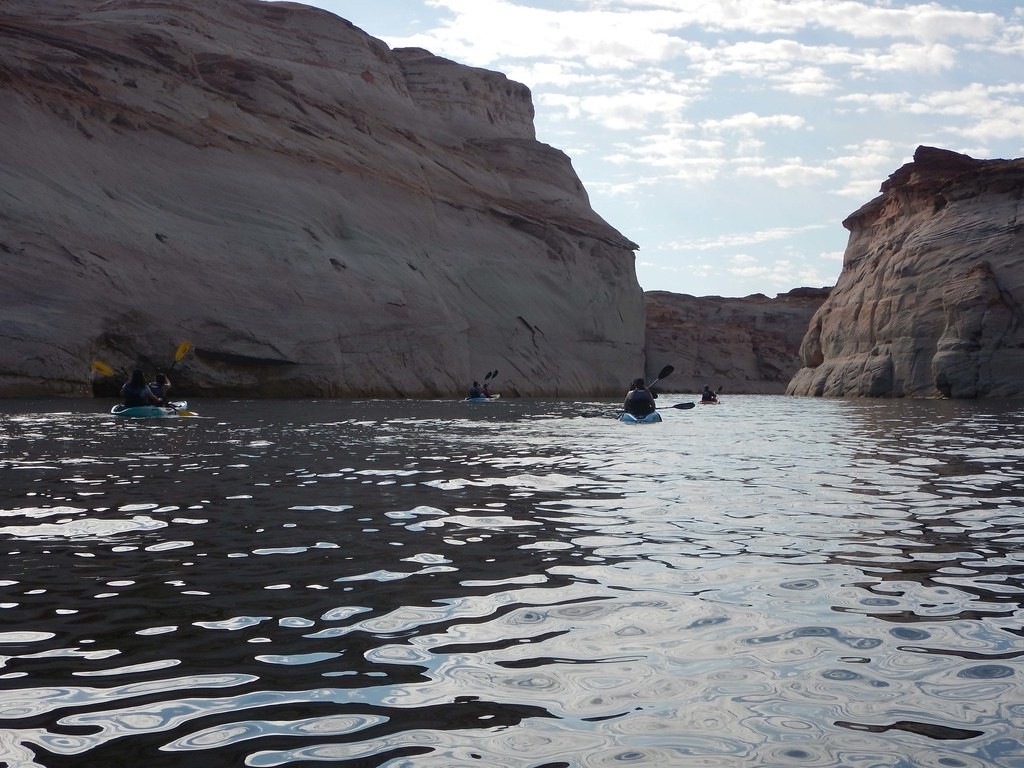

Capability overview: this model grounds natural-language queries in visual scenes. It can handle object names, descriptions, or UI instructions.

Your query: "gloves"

[158,398,163,402]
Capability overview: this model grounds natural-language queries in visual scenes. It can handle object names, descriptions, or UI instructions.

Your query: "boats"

[698,397,719,404]
[465,394,500,402]
[620,411,661,423]
[111,401,187,417]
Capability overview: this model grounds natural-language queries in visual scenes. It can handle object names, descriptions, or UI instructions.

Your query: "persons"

[120,370,163,408]
[148,374,171,401]
[701,384,716,401]
[624,378,655,419]
[470,382,481,398]
[481,384,489,398]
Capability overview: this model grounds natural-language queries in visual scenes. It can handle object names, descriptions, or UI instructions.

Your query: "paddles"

[483,371,493,384]
[580,402,696,418]
[715,385,723,393]
[170,340,192,372]
[490,369,499,384]
[90,359,195,417]
[616,364,675,419]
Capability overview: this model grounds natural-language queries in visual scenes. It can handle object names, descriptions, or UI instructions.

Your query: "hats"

[704,386,709,389]
